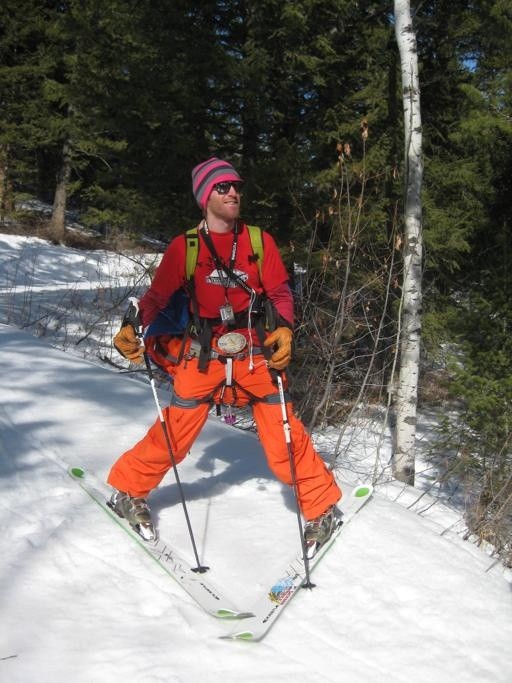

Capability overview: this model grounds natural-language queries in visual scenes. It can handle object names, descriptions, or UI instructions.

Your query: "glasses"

[213,181,244,193]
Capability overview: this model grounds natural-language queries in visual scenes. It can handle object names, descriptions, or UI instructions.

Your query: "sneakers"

[302,504,341,560]
[115,491,156,541]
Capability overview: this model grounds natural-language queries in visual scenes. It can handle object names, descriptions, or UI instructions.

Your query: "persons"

[108,156,344,559]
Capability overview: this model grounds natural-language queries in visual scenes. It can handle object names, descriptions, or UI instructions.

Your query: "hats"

[191,157,245,211]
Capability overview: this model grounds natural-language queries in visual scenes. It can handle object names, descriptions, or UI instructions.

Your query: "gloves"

[264,327,293,370]
[114,322,146,364]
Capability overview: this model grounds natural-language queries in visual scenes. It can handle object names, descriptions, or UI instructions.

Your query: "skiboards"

[65,465,373,641]
